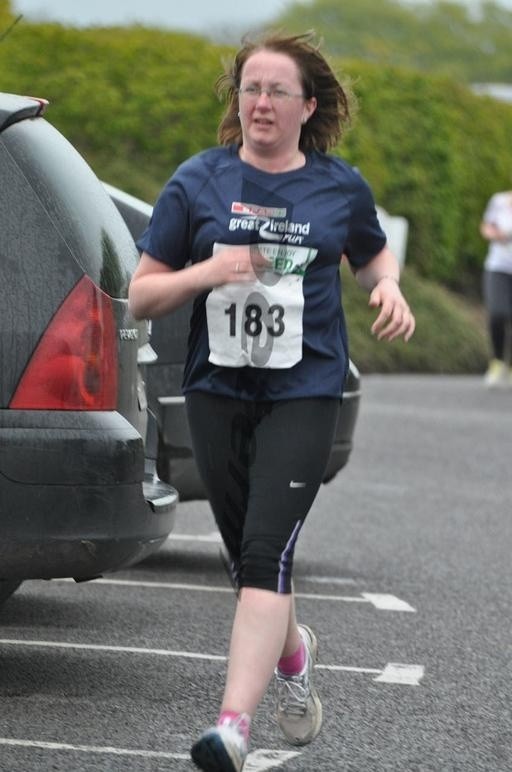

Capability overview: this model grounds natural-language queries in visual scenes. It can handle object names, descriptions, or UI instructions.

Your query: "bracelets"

[378,275,398,285]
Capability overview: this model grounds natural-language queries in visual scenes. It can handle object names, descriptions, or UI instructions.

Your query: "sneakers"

[191,713,251,772]
[275,623,323,746]
[485,358,506,384]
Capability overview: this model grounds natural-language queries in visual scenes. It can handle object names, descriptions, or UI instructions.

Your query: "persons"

[129,29,417,772]
[480,190,512,388]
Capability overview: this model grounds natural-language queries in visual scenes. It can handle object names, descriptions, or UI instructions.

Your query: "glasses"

[239,83,303,103]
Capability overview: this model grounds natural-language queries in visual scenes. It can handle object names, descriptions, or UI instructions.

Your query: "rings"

[235,262,240,273]
